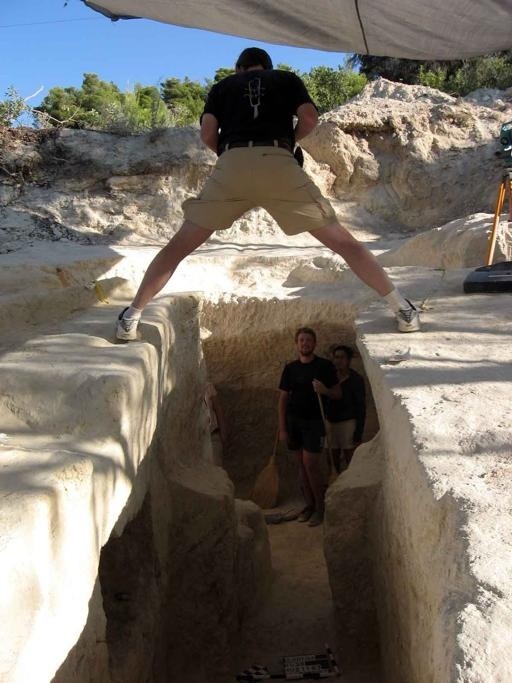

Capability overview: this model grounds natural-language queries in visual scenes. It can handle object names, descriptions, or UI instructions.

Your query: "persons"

[324,345,366,477]
[116,47,421,341]
[277,328,342,527]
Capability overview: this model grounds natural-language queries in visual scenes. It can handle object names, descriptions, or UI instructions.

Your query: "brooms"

[249,428,285,510]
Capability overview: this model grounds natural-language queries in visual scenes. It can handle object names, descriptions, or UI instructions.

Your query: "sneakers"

[306,512,324,527]
[395,296,422,333]
[297,507,314,522]
[114,306,139,341]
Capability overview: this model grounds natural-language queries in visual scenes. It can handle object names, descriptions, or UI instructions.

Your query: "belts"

[217,138,293,158]
